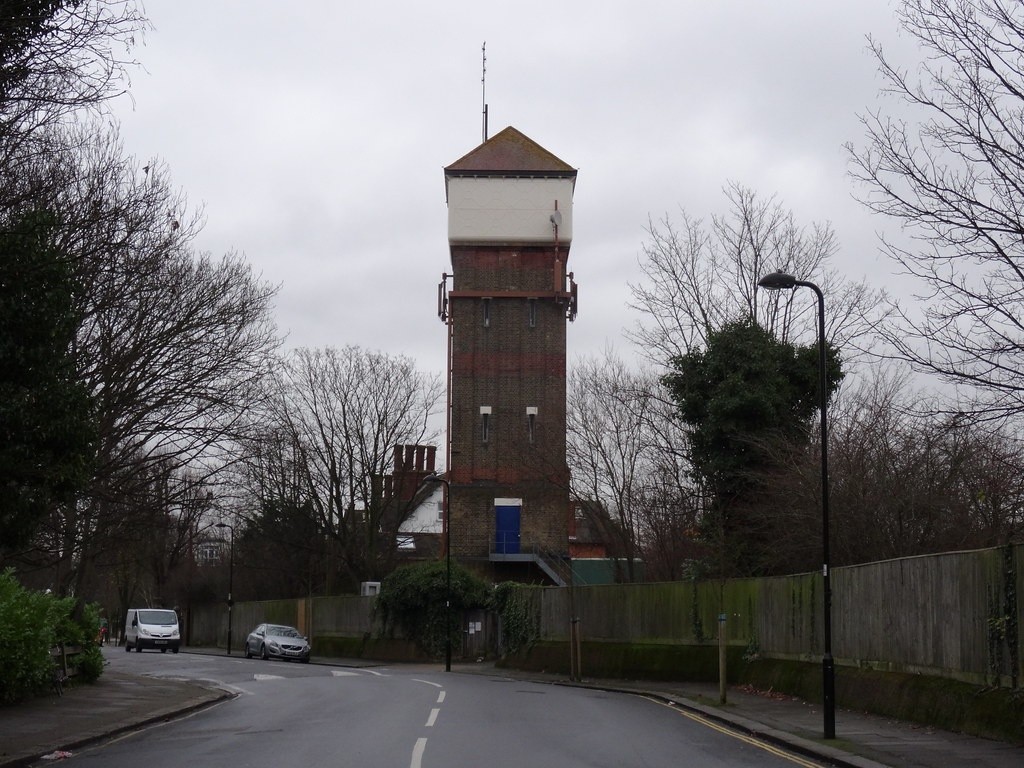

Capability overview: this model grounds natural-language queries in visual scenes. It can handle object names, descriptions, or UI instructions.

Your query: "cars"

[244,623,312,664]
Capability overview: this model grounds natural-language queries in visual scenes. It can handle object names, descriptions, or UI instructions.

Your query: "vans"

[124,609,184,654]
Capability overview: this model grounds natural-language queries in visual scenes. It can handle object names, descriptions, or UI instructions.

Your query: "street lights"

[216,523,234,655]
[423,474,452,673]
[758,271,837,740]
